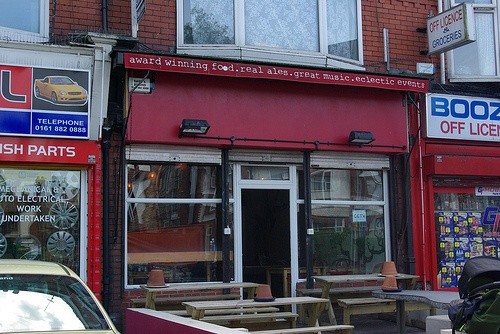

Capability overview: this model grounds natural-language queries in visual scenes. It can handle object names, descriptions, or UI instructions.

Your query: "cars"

[0,258,125,334]
[33,75,90,107]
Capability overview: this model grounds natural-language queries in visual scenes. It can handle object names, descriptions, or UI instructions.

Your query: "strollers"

[447,255,500,334]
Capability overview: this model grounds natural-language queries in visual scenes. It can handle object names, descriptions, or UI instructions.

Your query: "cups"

[147,270,165,285]
[255,285,273,298]
[383,276,398,290]
[381,261,398,275]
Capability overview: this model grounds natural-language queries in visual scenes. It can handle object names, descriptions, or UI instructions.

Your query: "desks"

[373,289,463,334]
[262,267,328,311]
[140,273,420,334]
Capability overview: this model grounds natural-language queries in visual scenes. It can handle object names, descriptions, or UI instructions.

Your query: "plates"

[378,274,400,277]
[382,288,402,292]
[253,296,276,301]
[144,284,170,287]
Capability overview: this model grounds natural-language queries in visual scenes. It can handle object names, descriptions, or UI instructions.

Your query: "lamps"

[178,119,210,134]
[349,131,375,145]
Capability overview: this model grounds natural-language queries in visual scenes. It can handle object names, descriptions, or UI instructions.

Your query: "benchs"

[337,298,437,325]
[204,313,298,329]
[296,286,383,294]
[131,294,240,307]
[159,307,279,315]
[249,325,354,334]
